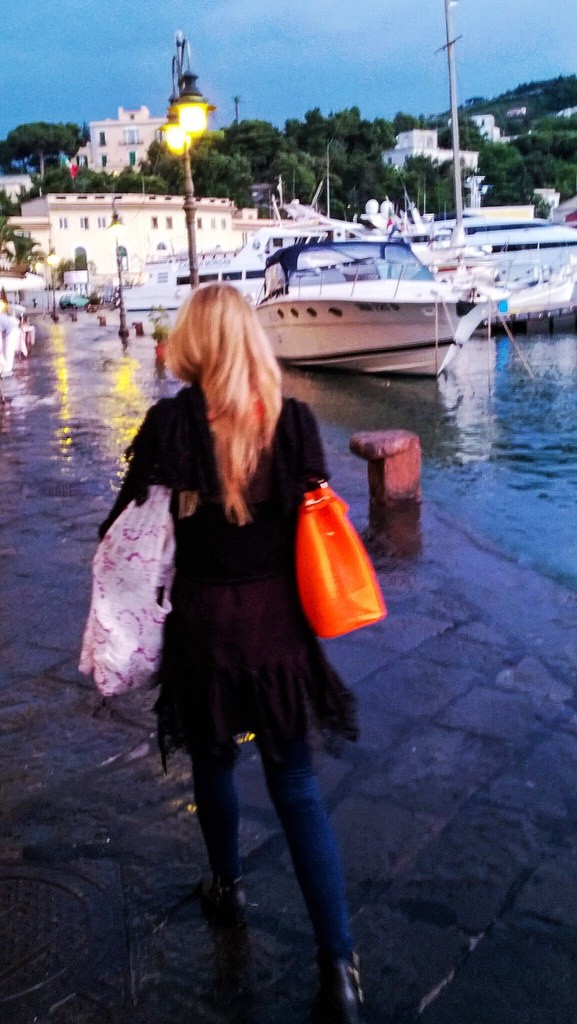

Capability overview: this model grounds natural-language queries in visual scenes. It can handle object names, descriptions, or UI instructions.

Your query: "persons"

[98,283,365,1024]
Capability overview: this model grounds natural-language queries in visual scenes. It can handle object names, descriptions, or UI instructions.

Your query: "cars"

[59,293,89,309]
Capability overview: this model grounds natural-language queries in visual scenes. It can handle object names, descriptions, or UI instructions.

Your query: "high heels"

[200,971,259,1024]
[310,956,359,1024]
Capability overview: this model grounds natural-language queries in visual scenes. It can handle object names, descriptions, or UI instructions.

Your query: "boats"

[128,217,577,327]
[253,234,498,379]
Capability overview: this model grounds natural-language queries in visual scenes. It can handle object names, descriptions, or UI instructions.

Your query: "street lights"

[166,29,202,288]
[233,96,240,122]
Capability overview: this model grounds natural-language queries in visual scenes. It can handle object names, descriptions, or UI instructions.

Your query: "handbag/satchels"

[78,485,176,696]
[290,398,385,640]
